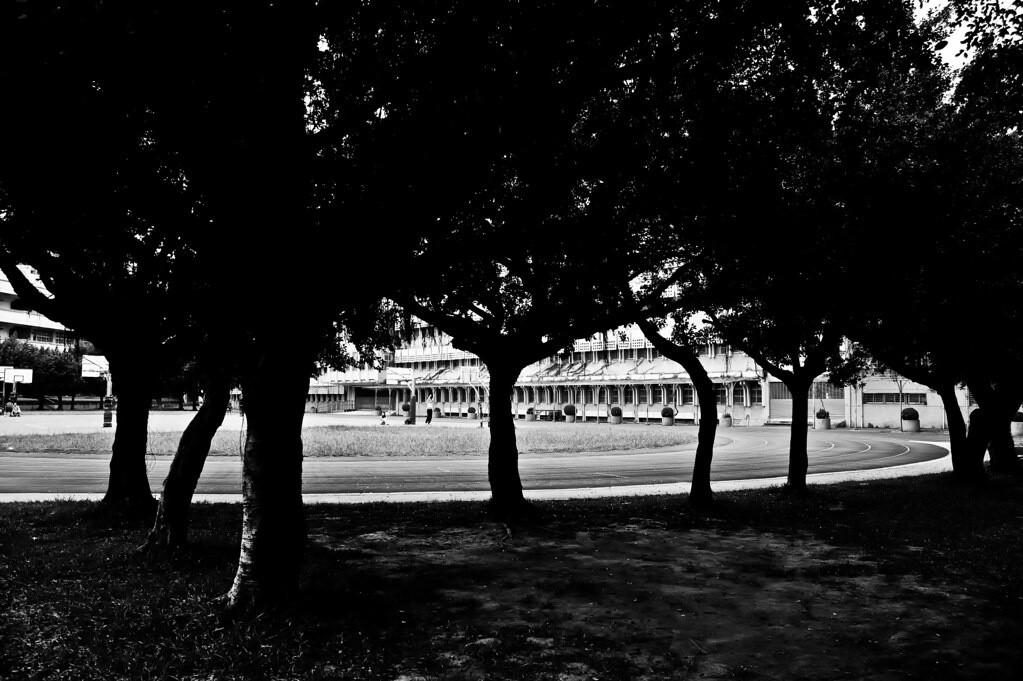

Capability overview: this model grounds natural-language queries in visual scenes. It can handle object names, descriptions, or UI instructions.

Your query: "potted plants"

[1011,411,1023,435]
[661,407,673,425]
[374,405,383,416]
[525,407,533,421]
[901,408,921,431]
[815,409,831,429]
[310,407,315,413]
[719,413,732,427]
[402,404,410,416]
[610,406,622,424]
[432,407,441,418]
[468,407,476,419]
[564,404,578,422]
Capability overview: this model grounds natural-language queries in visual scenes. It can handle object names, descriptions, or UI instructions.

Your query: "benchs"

[479,420,490,427]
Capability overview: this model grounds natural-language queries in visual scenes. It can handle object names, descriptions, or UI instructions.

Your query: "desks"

[534,409,562,421]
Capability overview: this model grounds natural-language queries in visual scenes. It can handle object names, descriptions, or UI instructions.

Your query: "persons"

[239,396,242,415]
[12,403,21,417]
[424,395,433,424]
[5,400,13,412]
[228,399,232,412]
